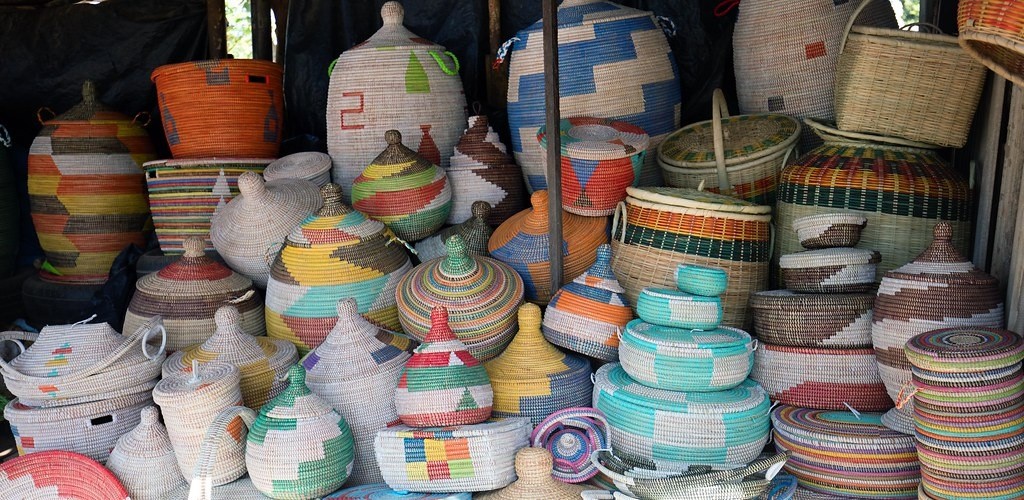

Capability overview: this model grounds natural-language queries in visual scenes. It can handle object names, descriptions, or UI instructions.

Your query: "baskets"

[612,0,1024,330]
